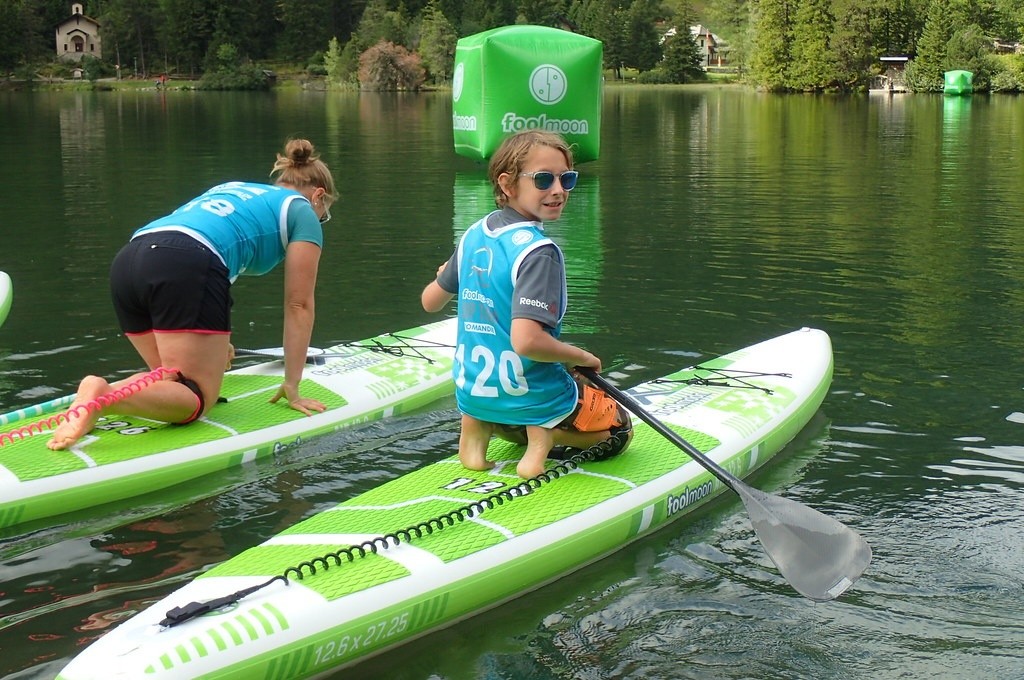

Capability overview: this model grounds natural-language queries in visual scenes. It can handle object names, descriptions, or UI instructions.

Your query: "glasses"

[319,197,332,225]
[519,171,578,192]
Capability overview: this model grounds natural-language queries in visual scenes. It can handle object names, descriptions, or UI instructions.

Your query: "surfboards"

[2,314,460,531]
[48,327,839,679]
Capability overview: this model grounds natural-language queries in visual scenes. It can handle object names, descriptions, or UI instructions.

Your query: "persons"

[48,140,340,450]
[421,128,632,481]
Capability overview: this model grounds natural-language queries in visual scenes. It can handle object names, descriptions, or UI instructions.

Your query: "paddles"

[574,366,872,602]
[233,345,321,361]
